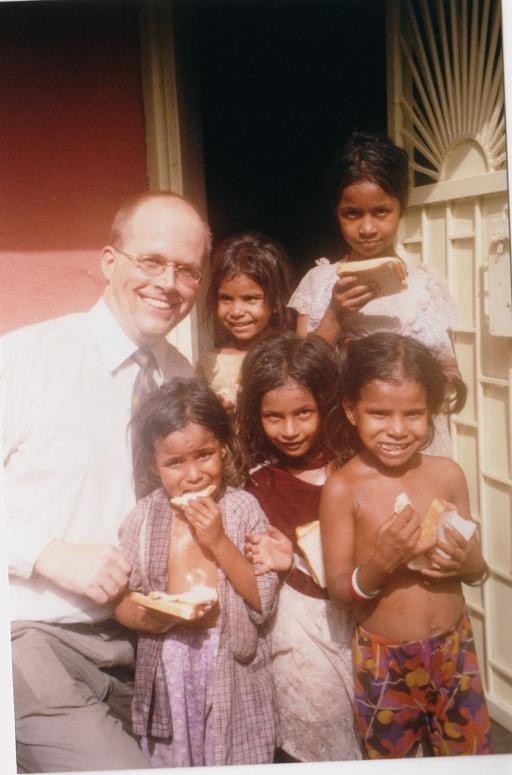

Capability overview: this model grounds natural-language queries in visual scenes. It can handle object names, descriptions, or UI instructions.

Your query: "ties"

[130,347,160,504]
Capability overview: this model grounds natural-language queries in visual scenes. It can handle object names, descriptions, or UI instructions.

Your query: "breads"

[394,491,413,513]
[169,484,217,507]
[336,257,408,300]
[406,499,476,573]
[129,588,218,620]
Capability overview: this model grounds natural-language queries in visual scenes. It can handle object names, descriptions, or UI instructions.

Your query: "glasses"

[119,248,205,287]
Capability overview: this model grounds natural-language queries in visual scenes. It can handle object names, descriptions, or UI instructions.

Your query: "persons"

[233,328,368,764]
[102,376,282,769]
[321,328,493,758]
[1,189,214,774]
[193,230,292,475]
[283,132,456,458]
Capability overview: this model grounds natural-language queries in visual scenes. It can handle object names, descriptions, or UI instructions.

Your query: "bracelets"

[463,559,490,589]
[351,566,382,600]
[345,571,364,603]
